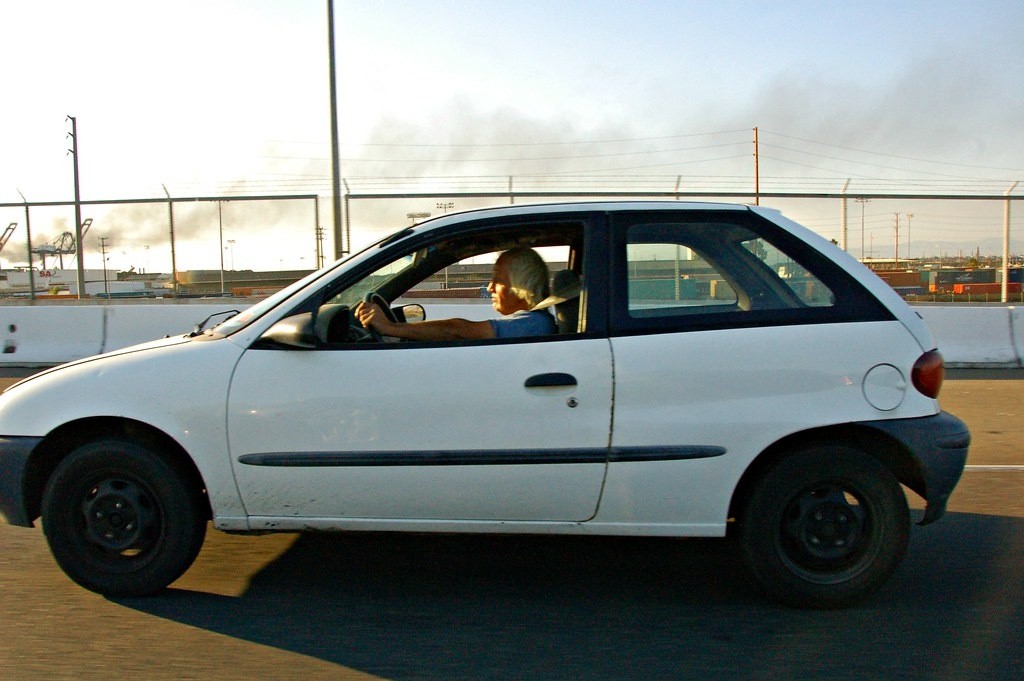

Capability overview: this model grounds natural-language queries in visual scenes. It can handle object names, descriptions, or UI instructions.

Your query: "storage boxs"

[232,269,1024,298]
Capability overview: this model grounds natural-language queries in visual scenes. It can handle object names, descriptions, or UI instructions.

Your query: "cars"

[0,200,972,612]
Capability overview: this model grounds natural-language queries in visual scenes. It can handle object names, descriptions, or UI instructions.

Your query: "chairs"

[552,270,579,331]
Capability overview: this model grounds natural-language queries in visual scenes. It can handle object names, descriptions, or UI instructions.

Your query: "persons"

[353,245,558,337]
